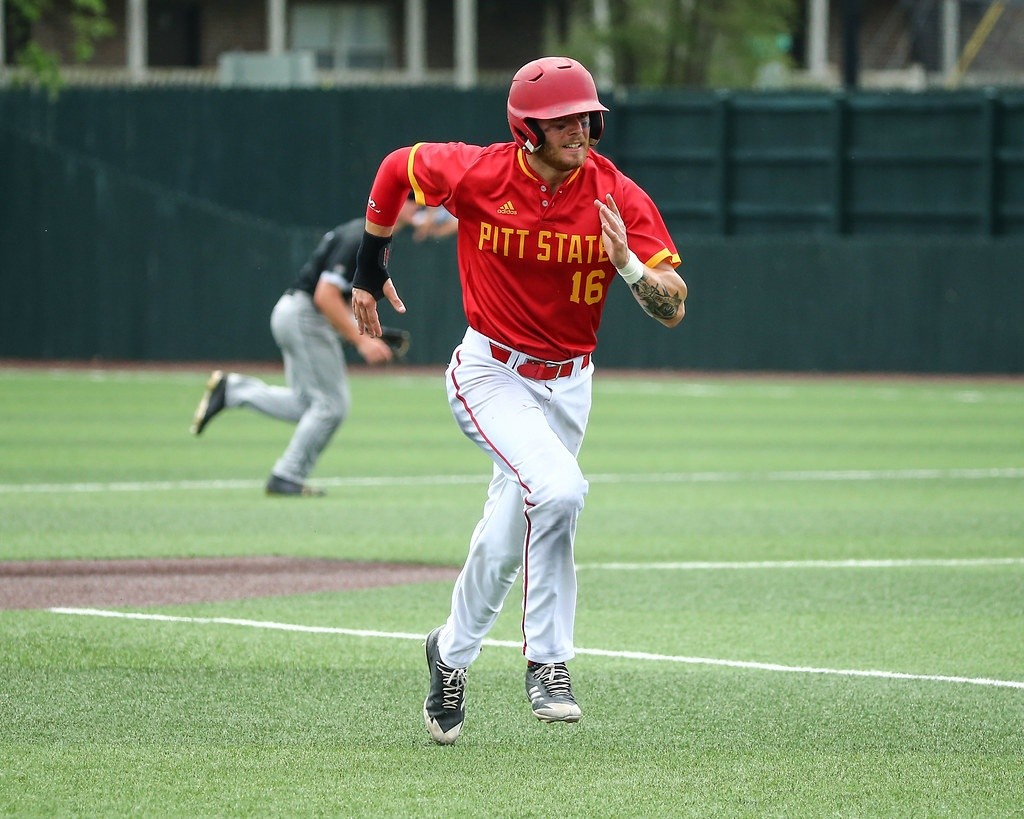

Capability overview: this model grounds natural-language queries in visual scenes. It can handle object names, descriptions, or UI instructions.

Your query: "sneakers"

[525,660,582,723]
[422,624,468,745]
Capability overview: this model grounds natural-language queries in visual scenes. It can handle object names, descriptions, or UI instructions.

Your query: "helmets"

[507,57,609,155]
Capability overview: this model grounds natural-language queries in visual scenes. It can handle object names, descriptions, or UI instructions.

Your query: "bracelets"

[611,250,643,284]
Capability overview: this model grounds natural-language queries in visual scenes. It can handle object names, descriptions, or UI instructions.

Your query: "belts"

[283,288,295,296]
[489,341,589,381]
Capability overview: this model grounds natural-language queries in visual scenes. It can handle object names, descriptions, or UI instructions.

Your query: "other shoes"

[192,370,227,434]
[266,474,324,497]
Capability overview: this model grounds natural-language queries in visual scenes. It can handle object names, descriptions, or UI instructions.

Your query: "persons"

[353,57,688,744]
[193,187,425,498]
